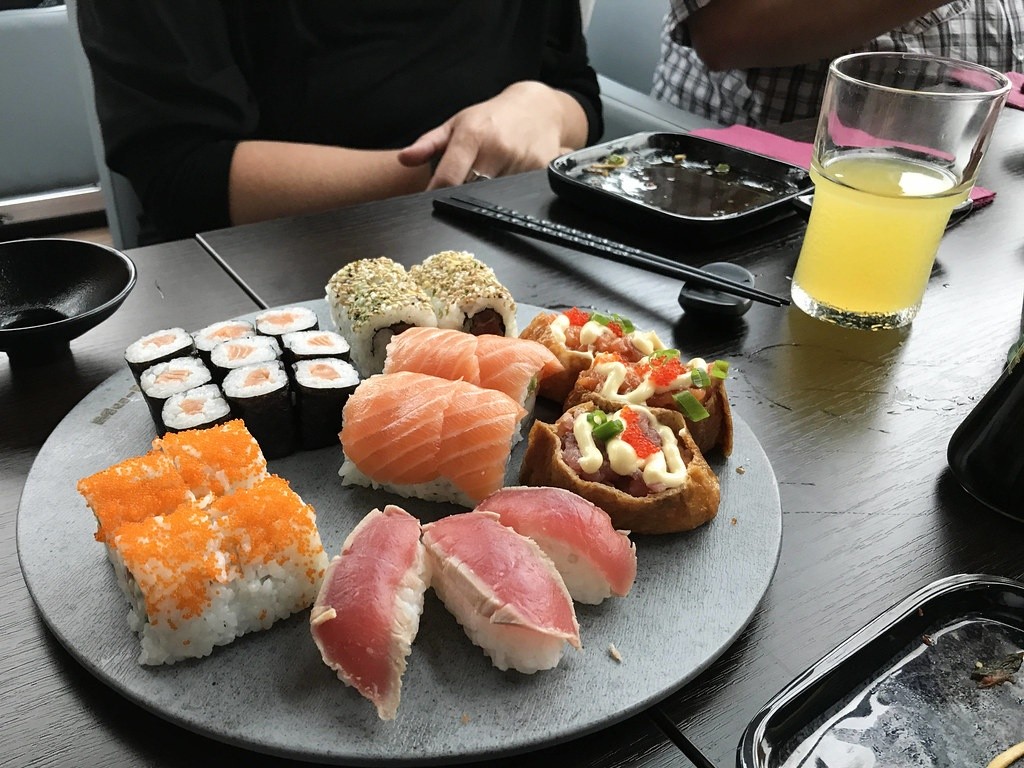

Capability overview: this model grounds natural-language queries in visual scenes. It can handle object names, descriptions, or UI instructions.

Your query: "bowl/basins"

[0,236,138,389]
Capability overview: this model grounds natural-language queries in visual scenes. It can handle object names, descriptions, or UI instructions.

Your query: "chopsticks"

[433,192,791,309]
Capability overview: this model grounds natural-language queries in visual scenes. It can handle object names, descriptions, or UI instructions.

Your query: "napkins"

[690,124,997,210]
[950,69,1024,110]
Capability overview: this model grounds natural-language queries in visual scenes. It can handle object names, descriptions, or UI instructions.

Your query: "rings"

[467,169,490,184]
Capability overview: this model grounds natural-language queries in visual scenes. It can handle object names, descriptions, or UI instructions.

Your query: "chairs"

[582,0,727,146]
[0,0,143,251]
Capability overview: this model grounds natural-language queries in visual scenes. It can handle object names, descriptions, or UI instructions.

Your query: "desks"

[0,83,1024,768]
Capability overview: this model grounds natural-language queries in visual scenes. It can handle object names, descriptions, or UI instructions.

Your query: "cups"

[790,51,1011,331]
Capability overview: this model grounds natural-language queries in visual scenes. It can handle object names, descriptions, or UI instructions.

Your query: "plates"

[735,573,1024,768]
[15,292,783,764]
[946,343,1024,523]
[548,130,816,243]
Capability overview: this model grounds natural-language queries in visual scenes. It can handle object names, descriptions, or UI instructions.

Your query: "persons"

[647,0,1024,132]
[75,0,604,248]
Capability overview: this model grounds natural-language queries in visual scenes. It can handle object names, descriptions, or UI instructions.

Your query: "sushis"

[75,249,636,722]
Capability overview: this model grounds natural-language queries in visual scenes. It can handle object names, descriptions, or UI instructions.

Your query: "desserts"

[516,304,733,537]
